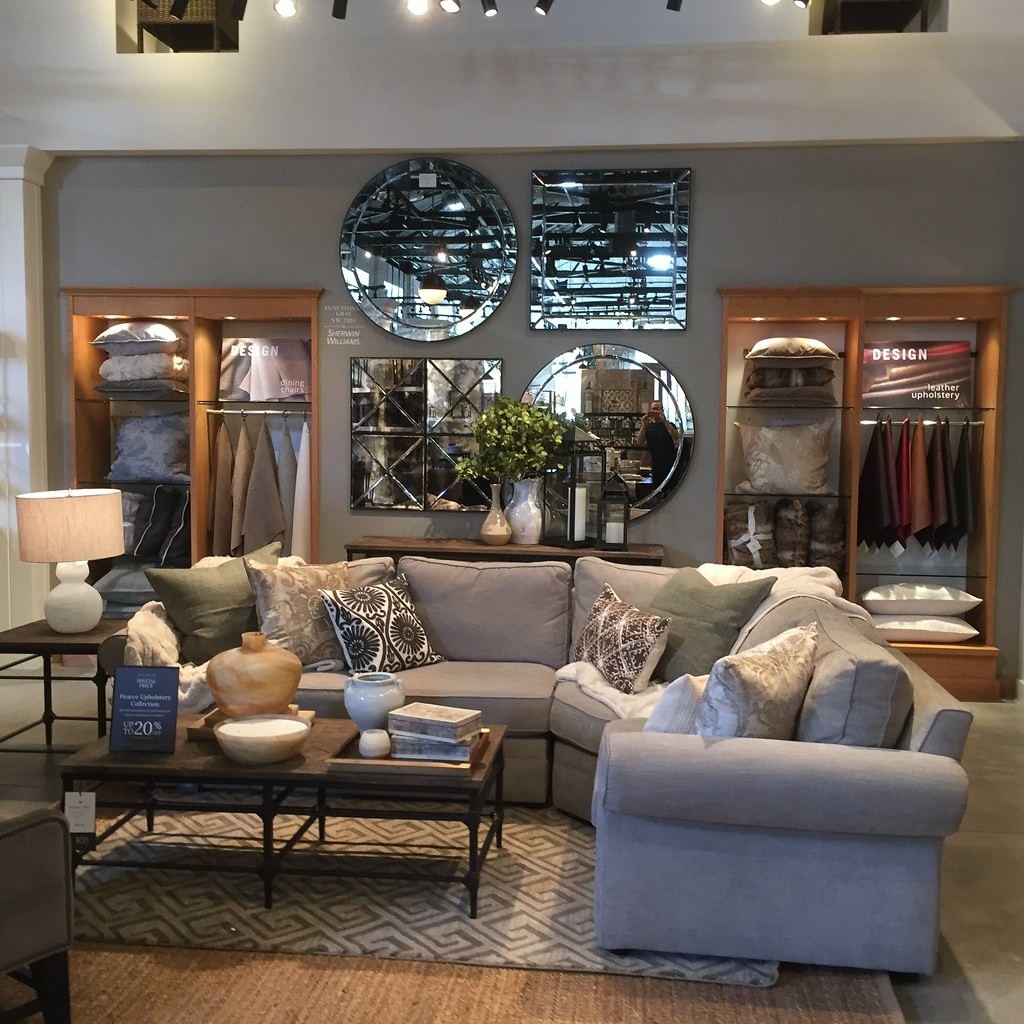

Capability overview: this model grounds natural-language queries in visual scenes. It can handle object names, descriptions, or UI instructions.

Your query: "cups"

[359,730,391,760]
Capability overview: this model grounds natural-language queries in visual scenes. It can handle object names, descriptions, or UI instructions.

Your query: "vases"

[479,477,544,544]
[206,631,302,717]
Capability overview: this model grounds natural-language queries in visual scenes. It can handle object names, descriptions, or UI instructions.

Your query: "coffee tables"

[56,712,511,920]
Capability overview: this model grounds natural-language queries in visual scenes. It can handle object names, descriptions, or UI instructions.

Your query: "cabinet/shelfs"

[58,286,326,564]
[714,285,1016,702]
[574,412,647,450]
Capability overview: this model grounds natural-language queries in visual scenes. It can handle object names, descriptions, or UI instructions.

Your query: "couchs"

[97,557,974,974]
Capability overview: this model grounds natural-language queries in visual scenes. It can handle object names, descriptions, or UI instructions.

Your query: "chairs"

[0,799,72,1024]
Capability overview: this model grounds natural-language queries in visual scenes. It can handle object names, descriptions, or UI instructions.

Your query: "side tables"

[0,617,131,755]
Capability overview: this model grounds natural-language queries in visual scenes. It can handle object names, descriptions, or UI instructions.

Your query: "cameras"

[647,411,660,418]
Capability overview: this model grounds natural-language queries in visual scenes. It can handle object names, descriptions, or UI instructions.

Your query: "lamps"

[645,223,651,232]
[14,488,124,633]
[600,223,608,233]
[793,0,810,8]
[168,0,684,21]
[576,351,593,390]
[418,267,448,305]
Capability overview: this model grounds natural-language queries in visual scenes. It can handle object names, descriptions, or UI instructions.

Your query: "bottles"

[344,672,405,736]
[206,632,301,717]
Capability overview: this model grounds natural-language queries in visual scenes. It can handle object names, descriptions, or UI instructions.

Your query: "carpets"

[69,786,780,987]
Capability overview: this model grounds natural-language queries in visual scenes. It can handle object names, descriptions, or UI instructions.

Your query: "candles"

[606,522,623,543]
[567,487,585,540]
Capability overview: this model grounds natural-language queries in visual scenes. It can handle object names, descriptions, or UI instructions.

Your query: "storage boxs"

[387,701,483,761]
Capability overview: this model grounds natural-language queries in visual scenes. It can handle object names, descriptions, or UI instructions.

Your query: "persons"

[638,400,679,490]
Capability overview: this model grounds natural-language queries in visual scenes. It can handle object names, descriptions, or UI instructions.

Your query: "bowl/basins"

[213,714,312,765]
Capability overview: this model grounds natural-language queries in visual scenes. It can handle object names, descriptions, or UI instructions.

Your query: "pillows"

[571,337,983,745]
[85,322,445,676]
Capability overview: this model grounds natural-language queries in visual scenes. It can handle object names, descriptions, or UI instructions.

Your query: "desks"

[344,535,664,567]
[587,475,653,502]
